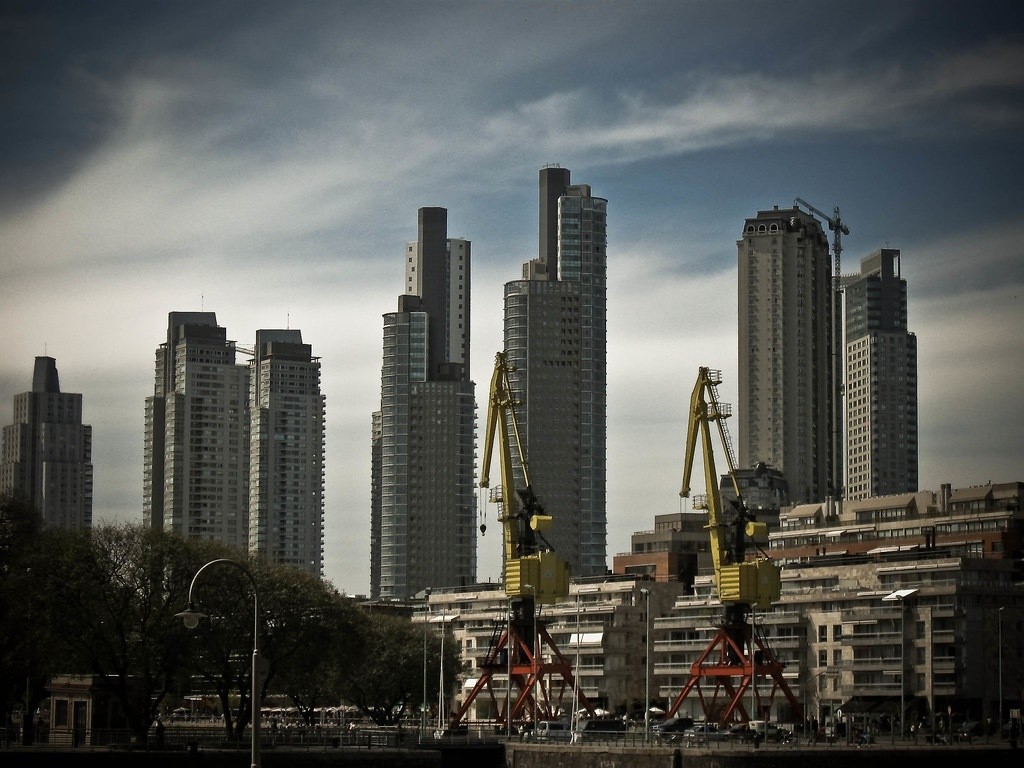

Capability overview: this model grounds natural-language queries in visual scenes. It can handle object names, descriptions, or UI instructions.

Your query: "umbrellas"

[576,706,666,720]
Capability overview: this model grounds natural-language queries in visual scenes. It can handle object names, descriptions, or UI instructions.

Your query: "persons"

[745,711,1019,749]
[517,722,524,742]
[270,718,406,747]
[154,719,167,747]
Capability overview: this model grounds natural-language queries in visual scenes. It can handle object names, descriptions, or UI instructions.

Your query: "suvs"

[651,717,694,736]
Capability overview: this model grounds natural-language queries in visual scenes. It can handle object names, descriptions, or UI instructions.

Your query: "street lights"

[423,593,430,737]
[175,557,264,768]
[524,583,537,741]
[750,601,757,722]
[895,595,905,742]
[997,605,1007,736]
[639,588,650,741]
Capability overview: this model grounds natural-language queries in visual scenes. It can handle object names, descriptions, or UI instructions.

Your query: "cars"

[685,725,719,736]
[724,724,750,734]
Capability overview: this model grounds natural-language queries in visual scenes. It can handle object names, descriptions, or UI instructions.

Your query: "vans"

[537,719,572,737]
[749,721,779,738]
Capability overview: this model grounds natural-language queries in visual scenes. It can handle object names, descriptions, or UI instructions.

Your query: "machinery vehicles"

[441,350,600,735]
[662,364,809,734]
